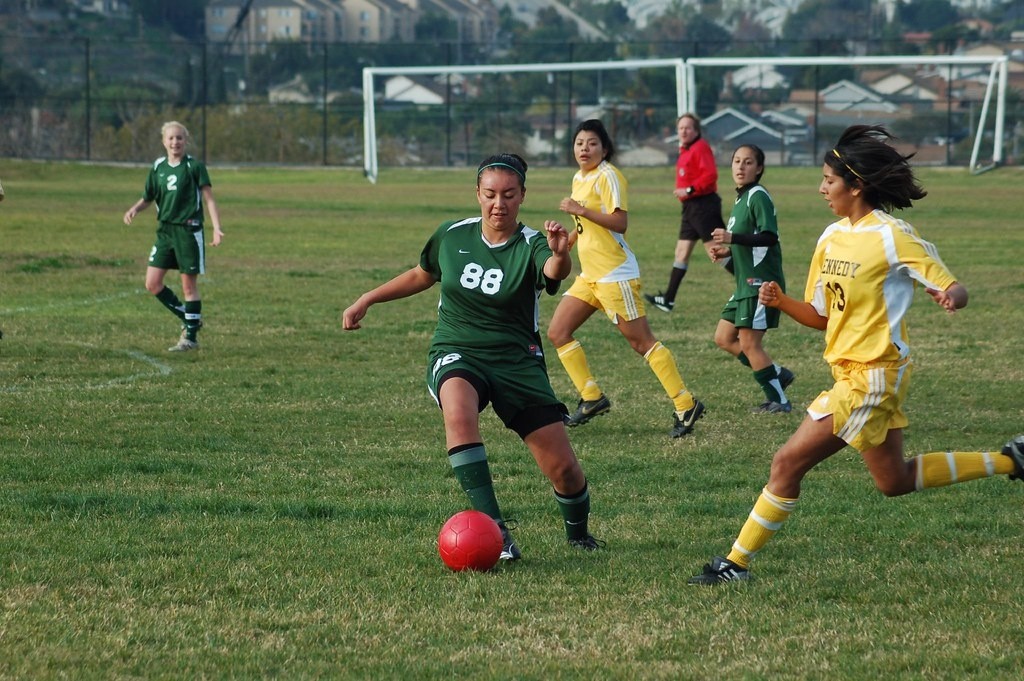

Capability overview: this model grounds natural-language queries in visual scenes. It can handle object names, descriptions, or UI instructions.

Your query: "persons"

[546,118,707,439]
[687,121,1024,585]
[643,113,734,313]
[342,154,608,563]
[708,144,795,413]
[123,121,225,351]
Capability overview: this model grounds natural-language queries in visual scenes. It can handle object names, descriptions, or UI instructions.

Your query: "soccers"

[438,509,503,571]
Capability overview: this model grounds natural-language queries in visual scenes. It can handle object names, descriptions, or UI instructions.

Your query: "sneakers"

[687,556,748,585]
[644,291,675,313]
[567,393,611,428]
[168,337,198,351]
[670,397,706,439]
[777,366,794,390]
[752,399,791,415]
[568,535,606,550]
[1001,436,1024,483]
[179,322,203,340]
[495,519,519,562]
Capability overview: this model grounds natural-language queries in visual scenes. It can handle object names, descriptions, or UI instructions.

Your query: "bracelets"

[686,186,691,193]
